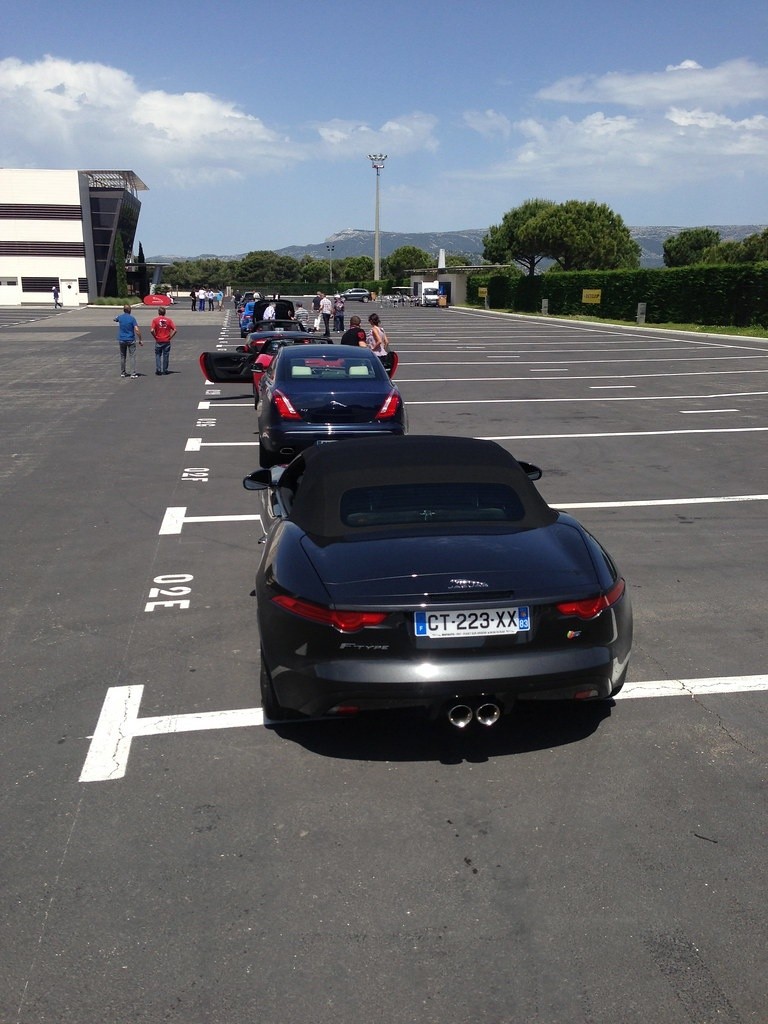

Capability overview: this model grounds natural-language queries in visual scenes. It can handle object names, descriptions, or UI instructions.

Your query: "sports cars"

[198,334,346,413]
[242,431,633,731]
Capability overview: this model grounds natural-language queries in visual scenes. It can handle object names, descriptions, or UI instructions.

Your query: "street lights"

[368,153,389,281]
[325,243,335,283]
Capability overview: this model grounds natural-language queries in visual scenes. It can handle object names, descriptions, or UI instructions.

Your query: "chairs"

[292,366,312,376]
[349,366,369,376]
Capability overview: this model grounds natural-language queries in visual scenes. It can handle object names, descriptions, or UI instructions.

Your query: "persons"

[252,291,280,302]
[52,286,62,309]
[190,287,243,312]
[113,305,143,379]
[341,316,367,347]
[263,302,310,332]
[150,307,178,376]
[311,292,345,338]
[366,313,389,369]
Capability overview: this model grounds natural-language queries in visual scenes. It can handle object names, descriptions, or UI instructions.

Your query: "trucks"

[417,278,440,308]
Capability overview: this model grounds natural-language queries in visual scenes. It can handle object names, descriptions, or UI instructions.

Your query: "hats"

[335,295,340,298]
[52,287,55,290]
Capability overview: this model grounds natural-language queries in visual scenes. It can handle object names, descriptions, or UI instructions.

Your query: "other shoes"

[131,375,138,379]
[121,373,127,377]
[163,371,168,374]
[155,371,161,375]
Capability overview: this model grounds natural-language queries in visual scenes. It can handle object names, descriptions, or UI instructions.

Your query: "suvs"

[334,288,373,302]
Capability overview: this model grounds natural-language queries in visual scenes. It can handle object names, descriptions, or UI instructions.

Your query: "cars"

[234,286,331,353]
[250,341,405,468]
